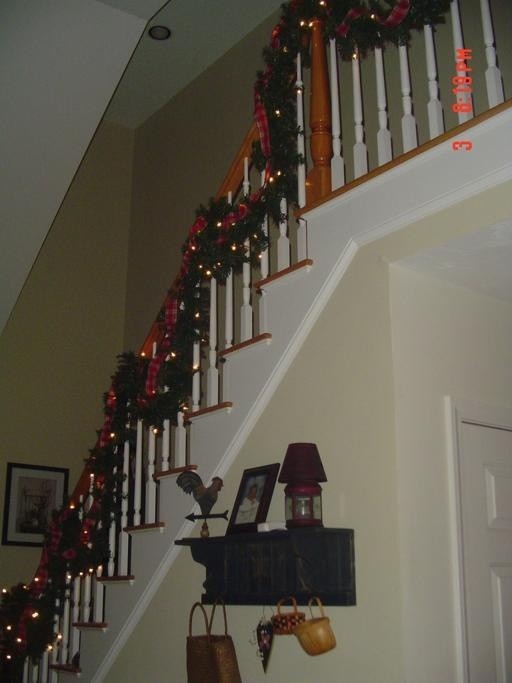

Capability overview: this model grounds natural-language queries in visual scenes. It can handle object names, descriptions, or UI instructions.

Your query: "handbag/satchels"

[271,596,305,634]
[186,599,242,683]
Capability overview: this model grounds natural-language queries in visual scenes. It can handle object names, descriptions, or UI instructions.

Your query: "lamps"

[278,438,327,525]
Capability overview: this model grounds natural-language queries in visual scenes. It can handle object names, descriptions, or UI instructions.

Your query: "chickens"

[173,466,225,516]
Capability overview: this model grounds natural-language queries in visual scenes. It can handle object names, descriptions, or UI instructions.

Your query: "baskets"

[294,597,336,656]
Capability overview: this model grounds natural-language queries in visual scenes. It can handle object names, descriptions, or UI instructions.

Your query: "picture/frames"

[3,460,70,547]
[225,460,280,532]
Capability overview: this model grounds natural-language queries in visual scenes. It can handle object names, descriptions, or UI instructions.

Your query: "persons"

[235,484,259,525]
